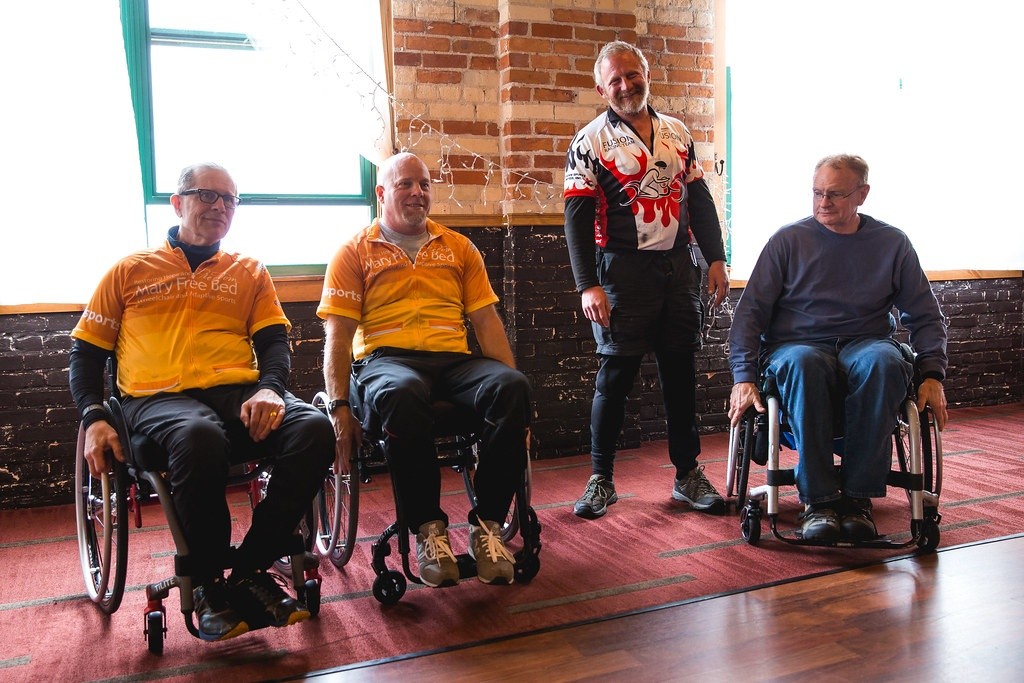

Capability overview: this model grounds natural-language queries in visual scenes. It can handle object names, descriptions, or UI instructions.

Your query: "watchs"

[327,399,351,414]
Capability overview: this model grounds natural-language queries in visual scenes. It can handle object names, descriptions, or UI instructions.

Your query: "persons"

[562,40,730,521]
[314,152,536,588]
[67,163,339,643]
[728,154,950,549]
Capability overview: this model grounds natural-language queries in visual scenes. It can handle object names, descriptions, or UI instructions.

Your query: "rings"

[269,411,277,417]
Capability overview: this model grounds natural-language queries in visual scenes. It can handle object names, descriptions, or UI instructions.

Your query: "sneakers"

[841,498,877,538]
[414,520,460,587]
[467,520,515,585]
[226,568,311,627]
[801,497,841,541]
[192,578,250,641]
[672,467,724,510]
[572,474,618,517]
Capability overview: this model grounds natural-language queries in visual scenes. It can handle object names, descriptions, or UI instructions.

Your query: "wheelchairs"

[725,341,943,553]
[76,345,323,661]
[311,365,542,605]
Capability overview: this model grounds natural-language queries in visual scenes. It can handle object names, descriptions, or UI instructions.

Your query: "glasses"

[179,189,242,209]
[813,186,860,202]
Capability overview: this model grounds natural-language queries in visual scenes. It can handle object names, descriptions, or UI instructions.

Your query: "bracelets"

[82,403,108,419]
